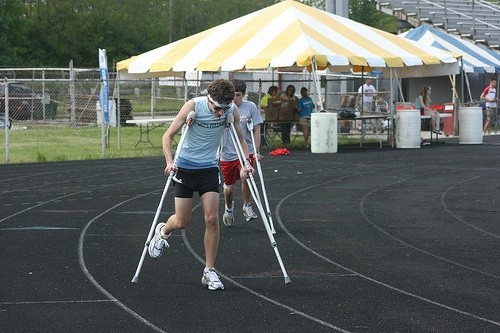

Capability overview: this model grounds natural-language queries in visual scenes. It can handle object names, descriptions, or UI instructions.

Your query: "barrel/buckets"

[396,110,421,149]
[311,112,338,154]
[459,106,483,144]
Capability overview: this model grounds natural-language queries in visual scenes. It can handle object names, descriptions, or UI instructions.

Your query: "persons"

[414,84,444,135]
[257,85,279,148]
[219,80,264,226]
[355,77,378,130]
[480,76,500,135]
[147,79,254,290]
[280,84,296,148]
[295,86,314,147]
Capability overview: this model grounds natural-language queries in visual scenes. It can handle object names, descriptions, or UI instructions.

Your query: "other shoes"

[433,129,443,135]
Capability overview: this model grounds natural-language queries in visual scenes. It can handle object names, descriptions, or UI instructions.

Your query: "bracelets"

[242,158,252,164]
[257,151,260,153]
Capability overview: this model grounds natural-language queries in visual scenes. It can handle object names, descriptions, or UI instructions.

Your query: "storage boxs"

[265,98,295,121]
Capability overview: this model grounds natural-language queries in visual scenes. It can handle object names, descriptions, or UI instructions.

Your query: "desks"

[337,116,389,149]
[126,118,178,148]
[263,121,299,148]
[421,114,452,144]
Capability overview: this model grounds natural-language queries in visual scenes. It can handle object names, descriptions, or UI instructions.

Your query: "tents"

[320,23,500,136]
[115,1,466,151]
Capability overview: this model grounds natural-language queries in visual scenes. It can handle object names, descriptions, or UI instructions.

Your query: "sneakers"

[242,203,257,219]
[148,222,172,259]
[201,268,224,290]
[223,201,235,227]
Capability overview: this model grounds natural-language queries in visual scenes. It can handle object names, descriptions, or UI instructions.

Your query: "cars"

[0,82,57,120]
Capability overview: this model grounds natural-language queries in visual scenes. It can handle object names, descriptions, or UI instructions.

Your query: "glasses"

[212,103,231,110]
